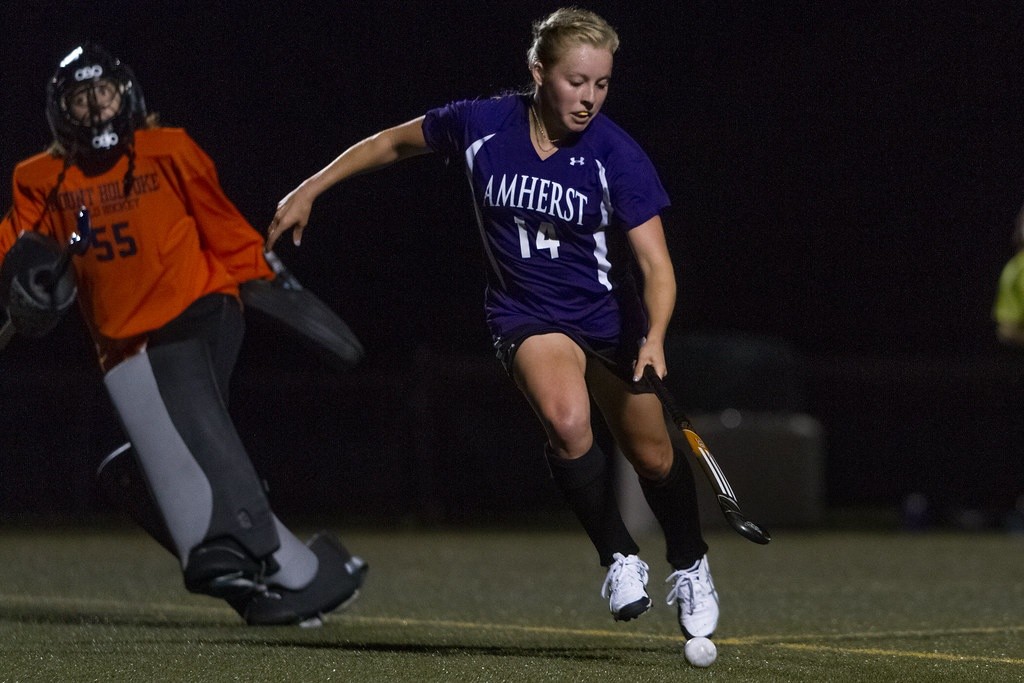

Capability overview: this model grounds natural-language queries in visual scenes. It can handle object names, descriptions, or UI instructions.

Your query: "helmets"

[45,42,149,177]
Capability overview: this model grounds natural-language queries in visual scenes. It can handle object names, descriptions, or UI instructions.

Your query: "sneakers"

[185,539,283,591]
[600,551,654,622]
[662,553,721,640]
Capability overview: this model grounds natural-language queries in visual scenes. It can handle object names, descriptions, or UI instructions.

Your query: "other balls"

[682,636,718,669]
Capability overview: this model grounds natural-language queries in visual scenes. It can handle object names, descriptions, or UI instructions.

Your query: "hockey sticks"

[0,203,92,353]
[617,315,775,547]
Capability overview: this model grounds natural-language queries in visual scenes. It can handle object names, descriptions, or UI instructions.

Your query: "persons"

[990,209,1024,344]
[0,41,368,627]
[265,6,720,640]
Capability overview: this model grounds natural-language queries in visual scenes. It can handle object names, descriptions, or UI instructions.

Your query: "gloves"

[8,264,86,339]
[240,249,366,363]
